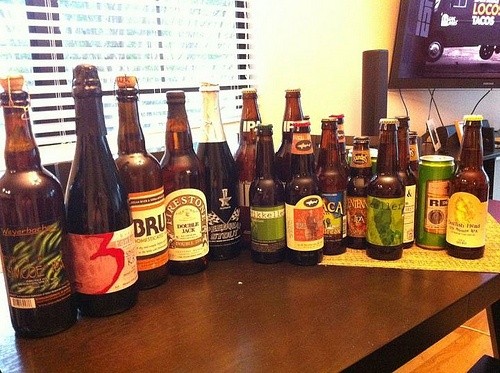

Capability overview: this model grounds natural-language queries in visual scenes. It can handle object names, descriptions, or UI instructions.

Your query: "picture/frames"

[426,119,442,152]
[454,120,465,144]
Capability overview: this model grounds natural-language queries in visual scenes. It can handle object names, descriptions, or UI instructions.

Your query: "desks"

[0,198,500,373]
[420,147,500,200]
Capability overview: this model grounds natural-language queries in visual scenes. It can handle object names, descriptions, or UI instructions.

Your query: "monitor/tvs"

[387,0,500,89]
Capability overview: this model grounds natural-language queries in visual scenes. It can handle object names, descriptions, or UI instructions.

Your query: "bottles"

[328,113,349,177]
[0,75,77,338]
[159,90,210,276]
[64,63,139,317]
[365,118,405,261]
[233,88,263,243]
[445,115,489,259]
[317,119,347,255]
[274,89,306,189]
[346,136,373,250]
[408,131,422,178]
[249,124,286,264]
[115,75,170,291]
[196,86,241,261]
[395,116,416,250]
[284,122,324,266]
